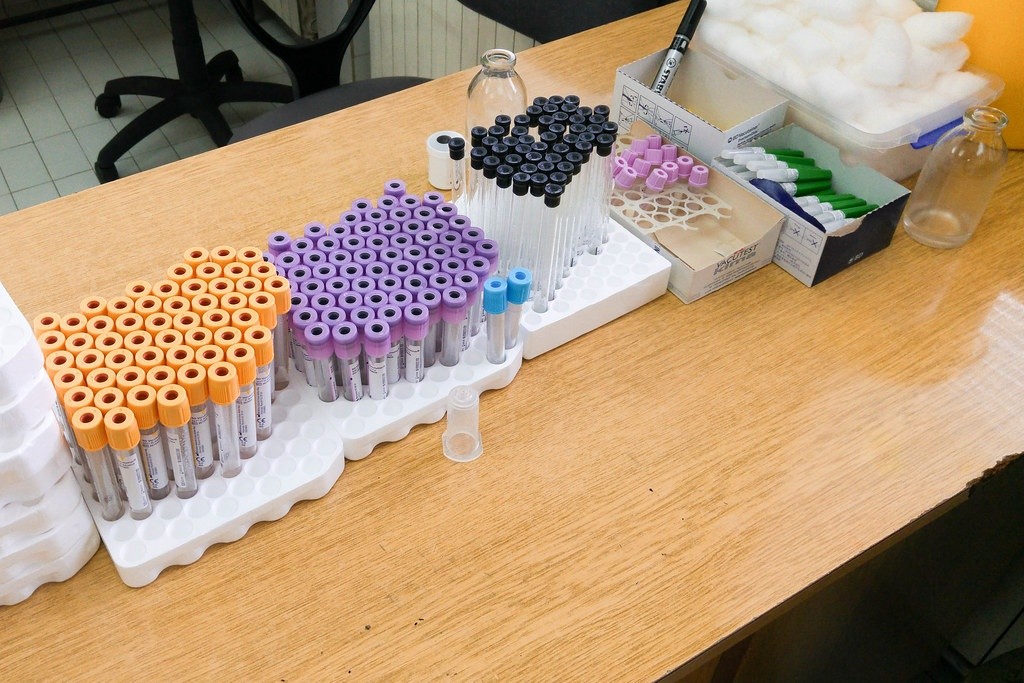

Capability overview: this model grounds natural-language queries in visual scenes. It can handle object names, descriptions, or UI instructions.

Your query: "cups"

[442,385,483,462]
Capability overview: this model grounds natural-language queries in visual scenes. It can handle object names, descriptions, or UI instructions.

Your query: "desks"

[0,0,1024,683]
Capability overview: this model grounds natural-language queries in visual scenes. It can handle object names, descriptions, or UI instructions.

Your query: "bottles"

[902,104,1010,250]
[465,49,528,145]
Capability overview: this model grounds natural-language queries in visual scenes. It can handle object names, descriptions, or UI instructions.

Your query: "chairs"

[92,0,435,193]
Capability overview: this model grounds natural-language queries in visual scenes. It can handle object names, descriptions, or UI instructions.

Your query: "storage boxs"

[608,40,1005,305]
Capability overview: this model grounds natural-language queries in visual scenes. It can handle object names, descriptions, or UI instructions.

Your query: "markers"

[648,0,708,96]
